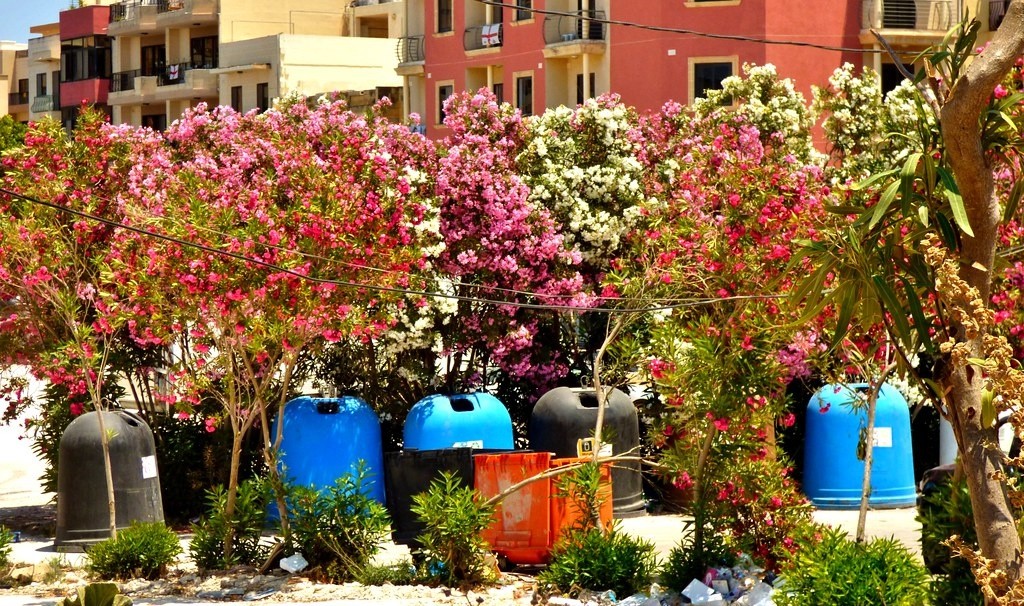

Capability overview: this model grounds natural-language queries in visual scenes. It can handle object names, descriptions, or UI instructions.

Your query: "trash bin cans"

[383,446,616,574]
